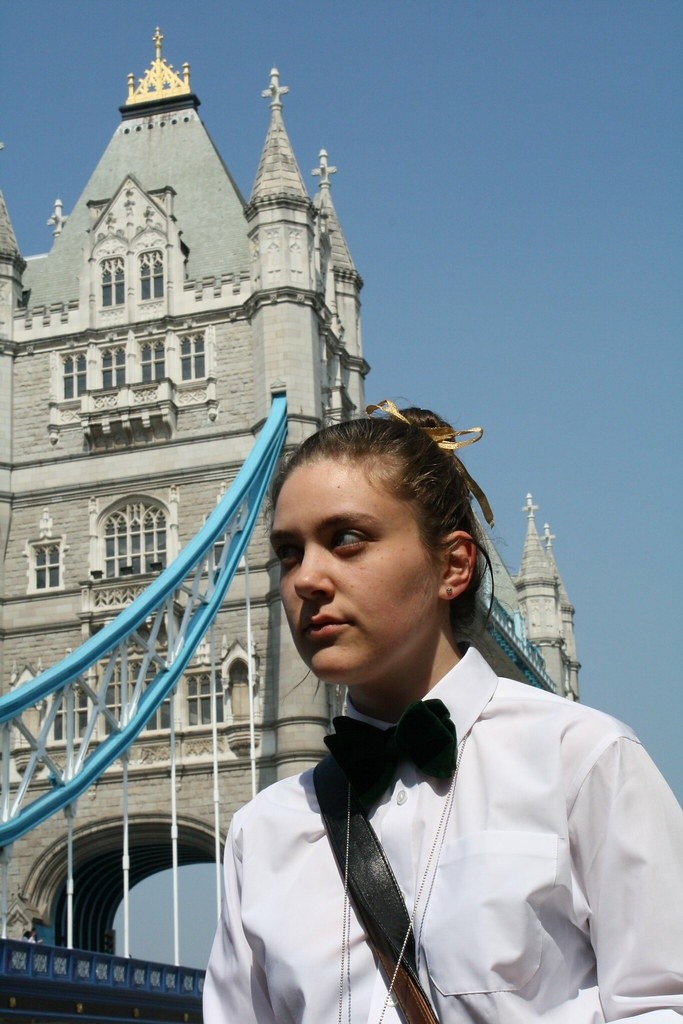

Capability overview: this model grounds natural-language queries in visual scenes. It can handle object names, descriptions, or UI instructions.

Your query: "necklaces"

[326,737,463,1024]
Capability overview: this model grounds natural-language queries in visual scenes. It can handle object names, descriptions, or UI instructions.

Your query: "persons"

[21,927,38,944]
[203,400,682,1024]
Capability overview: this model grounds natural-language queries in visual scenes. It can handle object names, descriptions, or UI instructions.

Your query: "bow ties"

[323,698,456,810]
